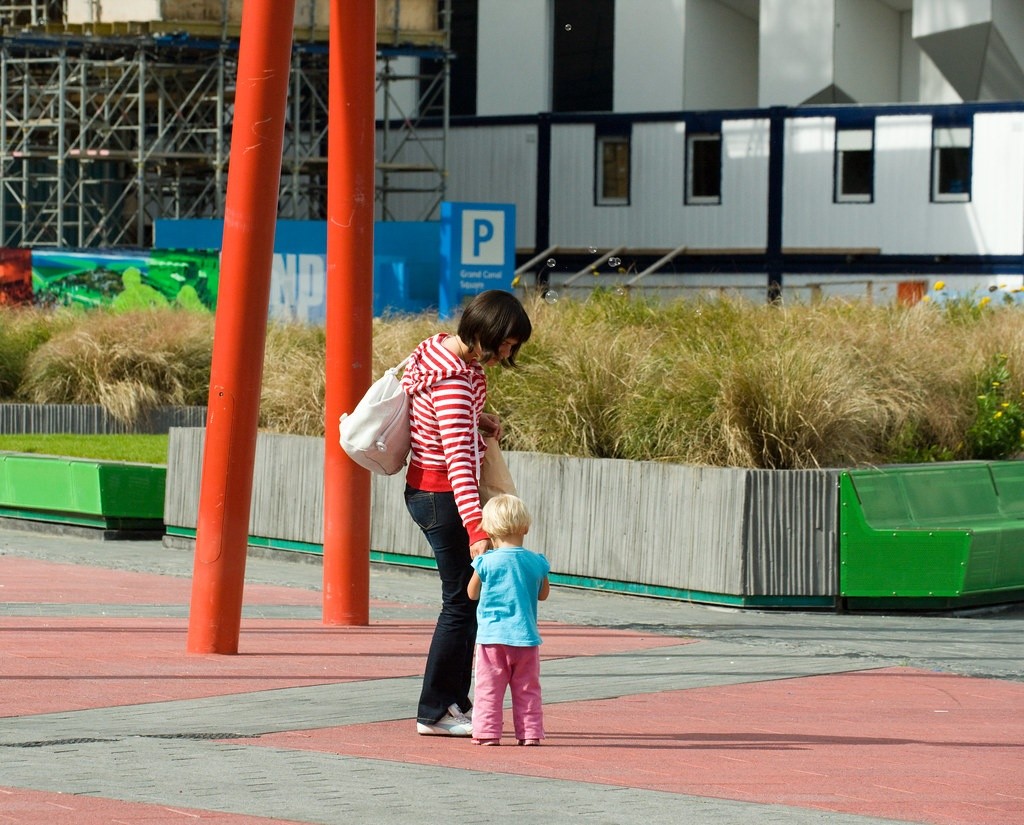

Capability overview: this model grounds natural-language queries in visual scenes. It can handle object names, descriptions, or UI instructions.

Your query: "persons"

[396,291,532,736]
[467,494,550,745]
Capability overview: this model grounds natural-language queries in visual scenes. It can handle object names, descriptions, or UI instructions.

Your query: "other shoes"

[471,738,499,746]
[517,739,540,745]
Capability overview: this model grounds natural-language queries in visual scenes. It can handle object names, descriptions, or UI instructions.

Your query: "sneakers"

[417,703,473,735]
[464,707,473,721]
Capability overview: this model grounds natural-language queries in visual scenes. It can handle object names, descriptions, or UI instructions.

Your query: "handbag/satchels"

[478,434,517,509]
[339,356,411,476]
